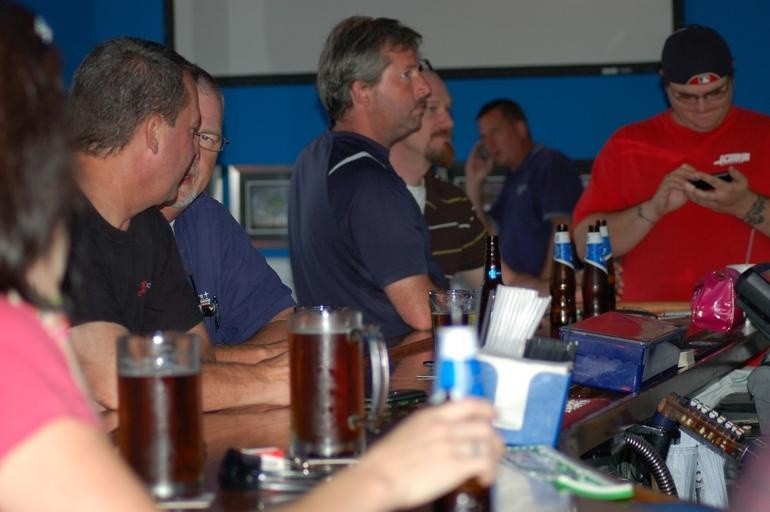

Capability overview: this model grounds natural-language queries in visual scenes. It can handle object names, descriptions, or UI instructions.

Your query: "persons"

[385,59,626,308]
[287,15,450,330]
[0,0,504,512]
[464,98,581,284]
[570,24,770,304]
[157,60,297,364]
[61,33,316,413]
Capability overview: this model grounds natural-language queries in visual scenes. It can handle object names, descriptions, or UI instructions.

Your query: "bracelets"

[637,204,657,226]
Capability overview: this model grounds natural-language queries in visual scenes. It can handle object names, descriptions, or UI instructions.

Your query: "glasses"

[195,132,229,152]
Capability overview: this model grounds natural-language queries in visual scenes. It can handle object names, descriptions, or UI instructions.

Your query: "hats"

[661,26,732,85]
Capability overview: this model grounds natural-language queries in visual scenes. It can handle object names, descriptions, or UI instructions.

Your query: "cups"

[428,289,472,347]
[115,329,207,498]
[287,303,391,472]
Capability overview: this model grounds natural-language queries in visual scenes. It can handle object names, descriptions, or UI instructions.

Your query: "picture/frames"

[240,173,291,240]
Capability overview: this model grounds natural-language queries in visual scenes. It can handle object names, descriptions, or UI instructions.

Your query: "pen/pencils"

[213,294,220,330]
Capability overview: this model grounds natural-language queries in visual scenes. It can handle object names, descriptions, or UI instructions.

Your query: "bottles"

[581,225,607,317]
[548,221,577,330]
[477,234,503,333]
[596,218,616,313]
[427,346,497,512]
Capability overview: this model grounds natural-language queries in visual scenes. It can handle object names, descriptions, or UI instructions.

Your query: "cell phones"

[688,171,735,192]
